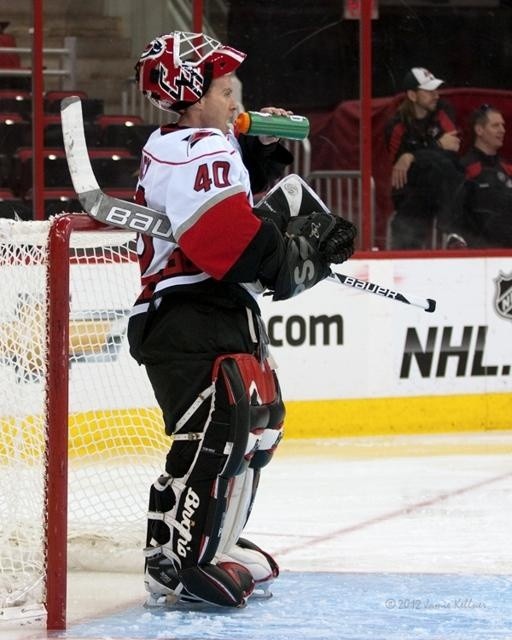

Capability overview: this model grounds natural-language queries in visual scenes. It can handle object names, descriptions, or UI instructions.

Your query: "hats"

[405,67,447,91]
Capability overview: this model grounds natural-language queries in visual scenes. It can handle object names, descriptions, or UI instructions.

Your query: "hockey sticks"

[60,96,437,314]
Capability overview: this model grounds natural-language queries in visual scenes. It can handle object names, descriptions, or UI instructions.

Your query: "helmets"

[135,31,248,117]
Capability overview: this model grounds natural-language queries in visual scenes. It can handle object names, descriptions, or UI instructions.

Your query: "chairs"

[0,18,161,222]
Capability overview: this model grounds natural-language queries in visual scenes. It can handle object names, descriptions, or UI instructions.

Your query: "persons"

[389,66,466,251]
[460,105,512,248]
[124,29,358,611]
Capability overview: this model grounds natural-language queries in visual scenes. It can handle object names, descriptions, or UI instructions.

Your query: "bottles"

[230,110,311,142]
